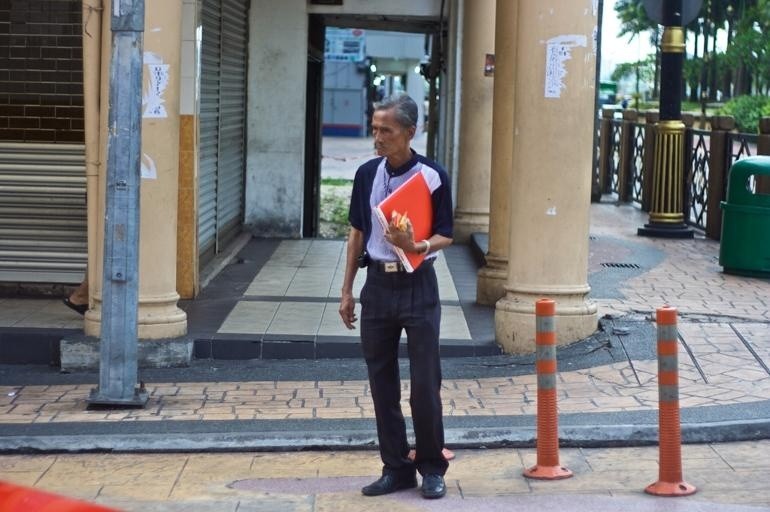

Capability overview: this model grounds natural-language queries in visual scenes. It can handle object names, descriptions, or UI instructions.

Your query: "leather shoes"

[421,472,446,497]
[362,472,418,494]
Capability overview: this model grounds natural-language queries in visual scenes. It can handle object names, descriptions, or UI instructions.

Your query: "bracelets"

[421,238,432,254]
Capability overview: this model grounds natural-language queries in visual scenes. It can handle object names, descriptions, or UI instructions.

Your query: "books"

[374,170,434,273]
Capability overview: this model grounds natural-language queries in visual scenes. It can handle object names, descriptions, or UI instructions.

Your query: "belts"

[379,262,405,274]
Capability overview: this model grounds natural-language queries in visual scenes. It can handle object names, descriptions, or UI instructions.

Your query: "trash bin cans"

[719,155,770,278]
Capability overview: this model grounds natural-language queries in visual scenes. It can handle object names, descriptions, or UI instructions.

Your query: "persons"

[337,91,453,497]
